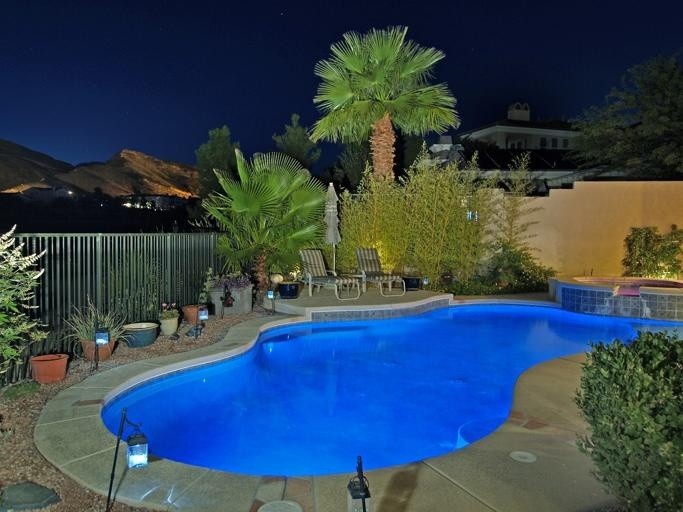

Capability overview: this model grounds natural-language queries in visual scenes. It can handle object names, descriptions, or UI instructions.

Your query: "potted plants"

[277,266,301,299]
[59,303,138,362]
[401,255,424,291]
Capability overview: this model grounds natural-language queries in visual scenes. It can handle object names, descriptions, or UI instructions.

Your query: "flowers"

[162,302,180,319]
[198,267,255,303]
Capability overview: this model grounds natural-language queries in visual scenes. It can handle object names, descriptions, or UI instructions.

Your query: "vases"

[207,284,255,316]
[121,322,159,348]
[29,354,69,384]
[182,304,200,324]
[160,317,180,336]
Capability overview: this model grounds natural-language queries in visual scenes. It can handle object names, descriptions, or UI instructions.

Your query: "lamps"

[220,286,235,319]
[265,280,276,315]
[91,320,111,369]
[346,456,371,512]
[193,303,208,339]
[106,408,149,512]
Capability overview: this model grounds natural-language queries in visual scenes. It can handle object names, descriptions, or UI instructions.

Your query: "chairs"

[299,249,361,301]
[355,247,407,298]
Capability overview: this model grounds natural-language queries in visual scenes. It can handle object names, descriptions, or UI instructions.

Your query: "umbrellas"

[323,182,341,276]
